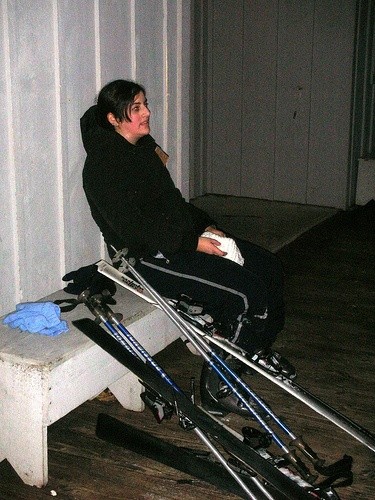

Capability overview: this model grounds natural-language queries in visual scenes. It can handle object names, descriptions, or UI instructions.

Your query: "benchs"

[0,285,209,488]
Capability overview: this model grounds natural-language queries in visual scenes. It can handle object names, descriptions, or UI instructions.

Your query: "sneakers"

[201,361,272,420]
[232,337,297,382]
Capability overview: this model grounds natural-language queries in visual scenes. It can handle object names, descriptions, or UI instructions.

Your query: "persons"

[80,79,297,421]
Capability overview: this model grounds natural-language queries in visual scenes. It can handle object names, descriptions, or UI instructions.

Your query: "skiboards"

[72,318,316,500]
[95,260,375,453]
[95,414,288,500]
